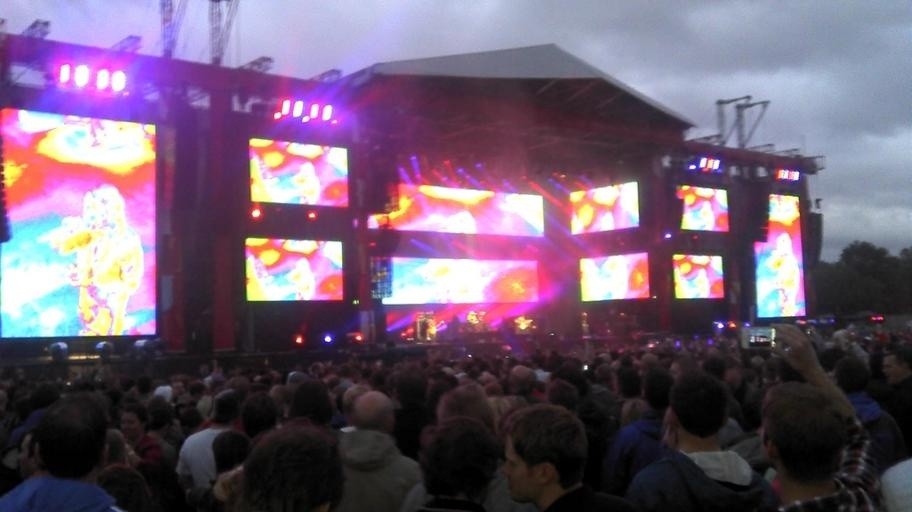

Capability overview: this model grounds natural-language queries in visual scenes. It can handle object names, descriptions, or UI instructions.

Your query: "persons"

[1,318,911,511]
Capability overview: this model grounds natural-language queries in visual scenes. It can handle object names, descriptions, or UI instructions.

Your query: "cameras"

[740,326,777,350]
